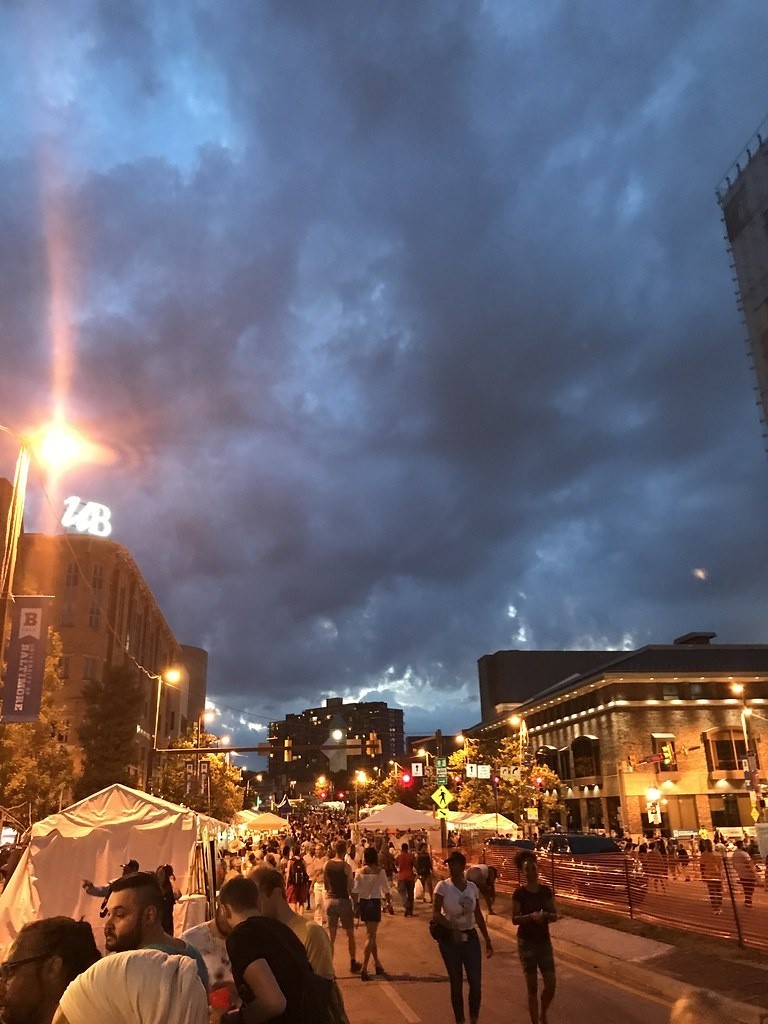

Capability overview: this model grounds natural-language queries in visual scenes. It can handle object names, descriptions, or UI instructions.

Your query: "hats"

[443,852,466,864]
[119,858,139,870]
[58,949,209,1024]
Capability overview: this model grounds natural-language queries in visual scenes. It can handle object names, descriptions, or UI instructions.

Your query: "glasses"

[0,954,52,983]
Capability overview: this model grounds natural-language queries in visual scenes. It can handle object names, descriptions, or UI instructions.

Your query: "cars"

[481,836,536,864]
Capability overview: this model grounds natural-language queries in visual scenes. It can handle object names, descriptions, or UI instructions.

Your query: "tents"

[233,801,518,854]
[0,784,230,974]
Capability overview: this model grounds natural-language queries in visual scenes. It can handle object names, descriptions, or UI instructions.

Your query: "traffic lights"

[493,775,501,789]
[454,776,461,791]
[402,773,411,788]
[535,777,542,788]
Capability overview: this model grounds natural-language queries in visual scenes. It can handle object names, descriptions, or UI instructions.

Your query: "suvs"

[531,831,649,903]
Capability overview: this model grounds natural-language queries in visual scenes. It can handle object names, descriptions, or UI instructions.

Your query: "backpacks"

[288,857,309,886]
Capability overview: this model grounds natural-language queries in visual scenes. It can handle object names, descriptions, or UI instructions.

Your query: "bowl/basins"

[207,986,231,1010]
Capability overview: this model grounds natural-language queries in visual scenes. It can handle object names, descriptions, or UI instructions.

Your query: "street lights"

[509,715,523,827]
[143,667,180,795]
[195,709,214,781]
[355,771,368,823]
[454,734,466,781]
[419,749,430,766]
[246,774,262,799]
[0,419,96,671]
[238,767,247,788]
[741,707,753,754]
[730,683,748,708]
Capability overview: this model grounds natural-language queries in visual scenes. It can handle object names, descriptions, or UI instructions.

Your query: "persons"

[0,804,768,1024]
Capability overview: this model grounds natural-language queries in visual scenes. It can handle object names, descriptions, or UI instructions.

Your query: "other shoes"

[388,906,394,915]
[376,967,384,975]
[361,974,372,981]
[405,909,410,917]
[714,909,721,914]
[350,962,362,971]
